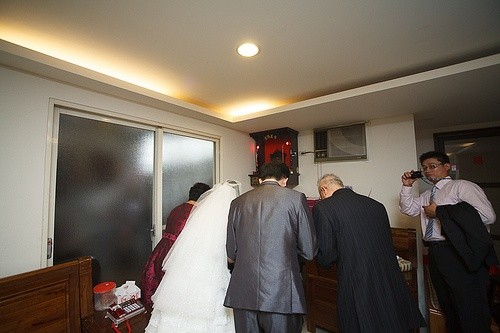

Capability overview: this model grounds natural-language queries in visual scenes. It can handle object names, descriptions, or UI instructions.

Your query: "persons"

[145,179,242,333]
[399,150,496,333]
[312,174,427,333]
[227,161,319,333]
[142,183,212,319]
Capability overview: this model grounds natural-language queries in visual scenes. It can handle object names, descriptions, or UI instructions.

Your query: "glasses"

[423,162,444,171]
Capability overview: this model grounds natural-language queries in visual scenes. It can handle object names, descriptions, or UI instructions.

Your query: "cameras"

[411,171,423,179]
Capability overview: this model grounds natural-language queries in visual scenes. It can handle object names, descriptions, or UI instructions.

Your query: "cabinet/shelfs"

[300,225,418,333]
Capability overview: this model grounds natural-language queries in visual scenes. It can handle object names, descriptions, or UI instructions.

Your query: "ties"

[425,185,436,239]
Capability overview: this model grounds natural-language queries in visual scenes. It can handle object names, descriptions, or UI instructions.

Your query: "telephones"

[106,298,146,333]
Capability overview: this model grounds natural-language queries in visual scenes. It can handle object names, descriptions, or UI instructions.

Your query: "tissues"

[115,280,143,302]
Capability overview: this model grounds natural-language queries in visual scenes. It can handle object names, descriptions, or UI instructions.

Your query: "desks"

[83,296,154,333]
